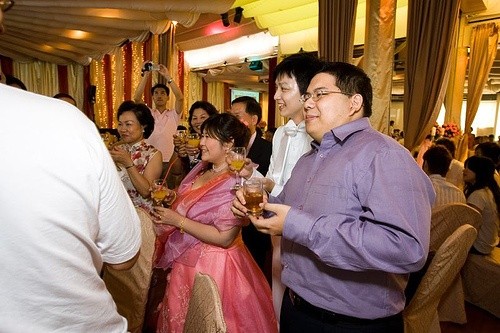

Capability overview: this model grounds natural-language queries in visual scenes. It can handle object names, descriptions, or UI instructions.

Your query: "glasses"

[298,89,354,104]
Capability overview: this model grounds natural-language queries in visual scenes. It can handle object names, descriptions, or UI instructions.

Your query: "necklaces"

[213,163,227,173]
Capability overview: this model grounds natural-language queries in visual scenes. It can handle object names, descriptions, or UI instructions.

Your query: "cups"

[100,133,111,149]
[243,180,264,217]
[175,129,186,146]
[186,133,200,156]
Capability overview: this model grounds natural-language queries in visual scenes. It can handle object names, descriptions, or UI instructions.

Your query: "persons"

[389,120,500,257]
[134,60,185,191]
[151,96,276,333]
[225,53,326,325]
[108,101,163,214]
[230,61,436,333]
[0,0,142,333]
[54,93,76,106]
[4,74,26,90]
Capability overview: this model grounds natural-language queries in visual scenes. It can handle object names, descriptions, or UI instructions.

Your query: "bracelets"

[127,164,134,168]
[168,78,173,84]
[180,218,185,234]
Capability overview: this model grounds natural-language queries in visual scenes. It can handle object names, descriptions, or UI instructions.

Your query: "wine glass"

[227,147,245,190]
[151,179,168,208]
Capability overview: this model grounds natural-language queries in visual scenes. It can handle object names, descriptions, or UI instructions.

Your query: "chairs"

[88,200,500,333]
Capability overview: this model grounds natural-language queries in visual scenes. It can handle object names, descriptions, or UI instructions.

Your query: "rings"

[158,216,161,220]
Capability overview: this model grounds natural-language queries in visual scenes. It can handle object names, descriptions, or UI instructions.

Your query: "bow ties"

[283,127,297,137]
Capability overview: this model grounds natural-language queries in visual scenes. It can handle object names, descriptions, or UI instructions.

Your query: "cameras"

[145,60,160,71]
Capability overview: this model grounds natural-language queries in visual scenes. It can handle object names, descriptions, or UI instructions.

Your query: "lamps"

[221,11,230,28]
[233,7,246,24]
